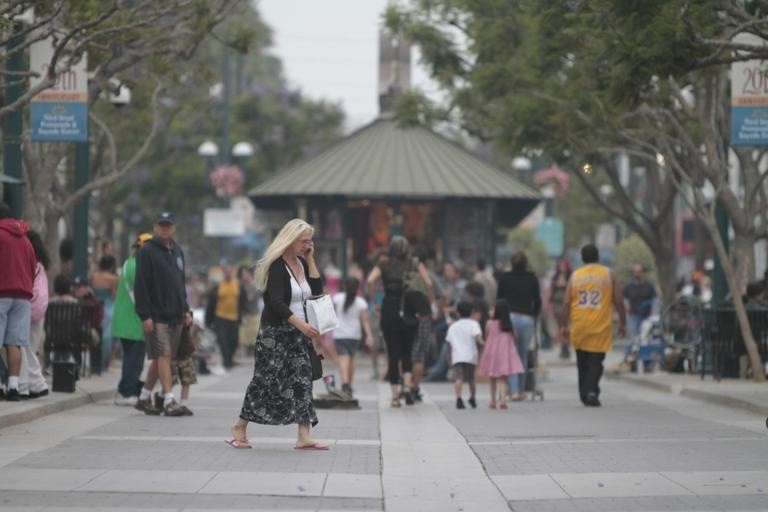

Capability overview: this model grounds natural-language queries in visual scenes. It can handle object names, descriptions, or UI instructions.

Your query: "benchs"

[702,310,768,381]
[44,302,93,380]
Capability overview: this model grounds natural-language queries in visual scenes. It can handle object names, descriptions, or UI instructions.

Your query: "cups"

[323,375,335,393]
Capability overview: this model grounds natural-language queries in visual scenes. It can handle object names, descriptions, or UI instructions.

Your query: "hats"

[155,213,175,225]
[135,233,152,246]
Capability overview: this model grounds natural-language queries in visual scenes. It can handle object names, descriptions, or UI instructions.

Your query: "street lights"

[198,136,254,212]
[76,75,129,283]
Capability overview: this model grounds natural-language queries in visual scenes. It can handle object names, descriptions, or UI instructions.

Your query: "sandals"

[390,385,420,406]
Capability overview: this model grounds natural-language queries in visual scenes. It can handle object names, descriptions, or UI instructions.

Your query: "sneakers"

[457,401,464,408]
[469,398,476,408]
[491,392,529,409]
[587,394,601,405]
[1,386,48,401]
[113,388,193,416]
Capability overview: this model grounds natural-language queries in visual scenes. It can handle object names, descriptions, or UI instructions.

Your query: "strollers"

[506,316,543,403]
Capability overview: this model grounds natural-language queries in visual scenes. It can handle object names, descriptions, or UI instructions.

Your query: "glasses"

[298,239,313,244]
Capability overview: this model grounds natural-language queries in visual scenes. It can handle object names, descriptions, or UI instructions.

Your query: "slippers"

[294,442,328,449]
[225,439,251,448]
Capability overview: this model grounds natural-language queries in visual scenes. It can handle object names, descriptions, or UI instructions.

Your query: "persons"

[226,218,332,449]
[324,231,627,407]
[624,261,712,372]
[0,201,264,416]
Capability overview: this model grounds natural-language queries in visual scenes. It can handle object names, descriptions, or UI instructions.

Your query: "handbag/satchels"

[308,343,324,380]
[306,294,338,335]
[403,256,430,314]
